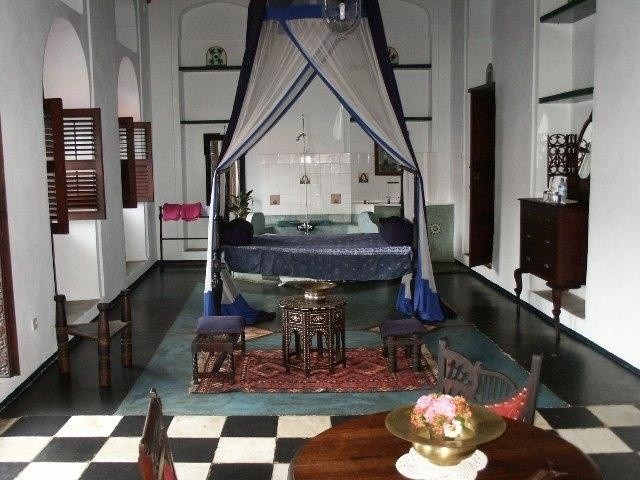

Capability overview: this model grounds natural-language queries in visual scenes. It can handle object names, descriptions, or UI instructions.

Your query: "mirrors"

[576,111,593,185]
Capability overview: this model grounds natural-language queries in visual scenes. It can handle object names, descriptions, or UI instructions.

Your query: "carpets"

[237,325,273,343]
[189,344,437,393]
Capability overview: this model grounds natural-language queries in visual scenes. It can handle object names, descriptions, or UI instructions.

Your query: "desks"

[277,295,346,377]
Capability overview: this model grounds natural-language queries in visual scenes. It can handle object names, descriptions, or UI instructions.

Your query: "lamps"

[321,0,362,39]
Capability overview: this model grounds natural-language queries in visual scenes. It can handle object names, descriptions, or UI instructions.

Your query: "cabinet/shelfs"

[514,197,589,328]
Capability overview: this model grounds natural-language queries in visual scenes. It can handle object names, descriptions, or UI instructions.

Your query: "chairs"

[137,387,178,480]
[437,338,544,426]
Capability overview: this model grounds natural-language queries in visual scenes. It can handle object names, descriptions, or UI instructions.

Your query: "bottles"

[543,191,550,201]
[558,178,567,202]
[551,192,558,202]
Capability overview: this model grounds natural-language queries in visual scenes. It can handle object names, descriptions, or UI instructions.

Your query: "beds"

[211,164,420,320]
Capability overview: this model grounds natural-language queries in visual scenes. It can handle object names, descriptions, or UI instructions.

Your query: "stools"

[190,316,246,386]
[378,319,425,373]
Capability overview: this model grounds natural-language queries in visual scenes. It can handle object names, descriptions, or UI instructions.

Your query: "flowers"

[409,392,472,440]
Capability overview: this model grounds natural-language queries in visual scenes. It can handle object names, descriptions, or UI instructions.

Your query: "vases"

[385,397,507,467]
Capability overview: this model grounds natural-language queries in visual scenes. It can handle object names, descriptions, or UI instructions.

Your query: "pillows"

[380,216,413,246]
[222,218,253,245]
